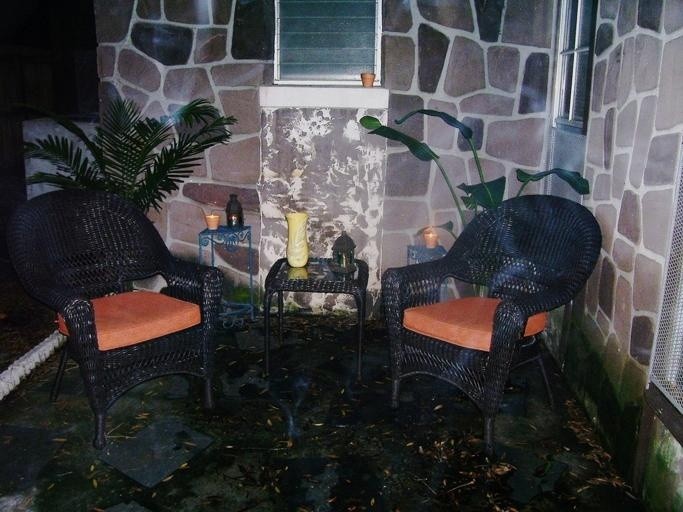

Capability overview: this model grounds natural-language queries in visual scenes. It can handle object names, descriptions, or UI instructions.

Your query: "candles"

[425,233,438,248]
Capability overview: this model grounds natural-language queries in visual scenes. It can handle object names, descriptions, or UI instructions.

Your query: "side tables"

[265,258,369,381]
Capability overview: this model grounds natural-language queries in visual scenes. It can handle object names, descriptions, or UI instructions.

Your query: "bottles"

[226,194,243,232]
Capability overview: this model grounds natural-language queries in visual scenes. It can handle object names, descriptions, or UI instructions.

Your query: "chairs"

[382,194,602,459]
[8,190,225,449]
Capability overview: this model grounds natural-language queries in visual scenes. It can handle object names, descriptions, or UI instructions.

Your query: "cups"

[424,233,439,249]
[361,73,375,88]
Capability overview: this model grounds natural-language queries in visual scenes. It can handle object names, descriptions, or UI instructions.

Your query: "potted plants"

[361,111,589,364]
[15,97,238,366]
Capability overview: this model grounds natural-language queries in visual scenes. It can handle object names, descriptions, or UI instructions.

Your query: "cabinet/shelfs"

[198,225,254,329]
[407,245,448,302]
